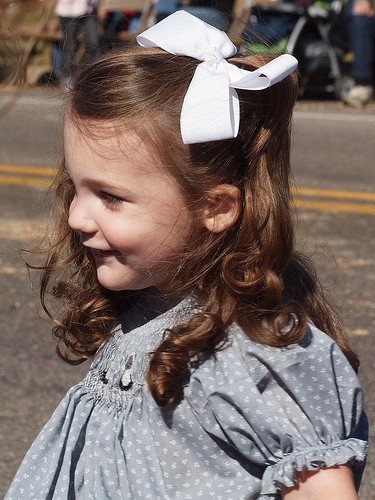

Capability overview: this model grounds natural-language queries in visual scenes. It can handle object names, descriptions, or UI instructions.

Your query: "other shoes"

[61,76,73,94]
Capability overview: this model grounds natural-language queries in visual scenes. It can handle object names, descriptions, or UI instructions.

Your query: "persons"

[0,10,368,500]
[53,0,374,107]
